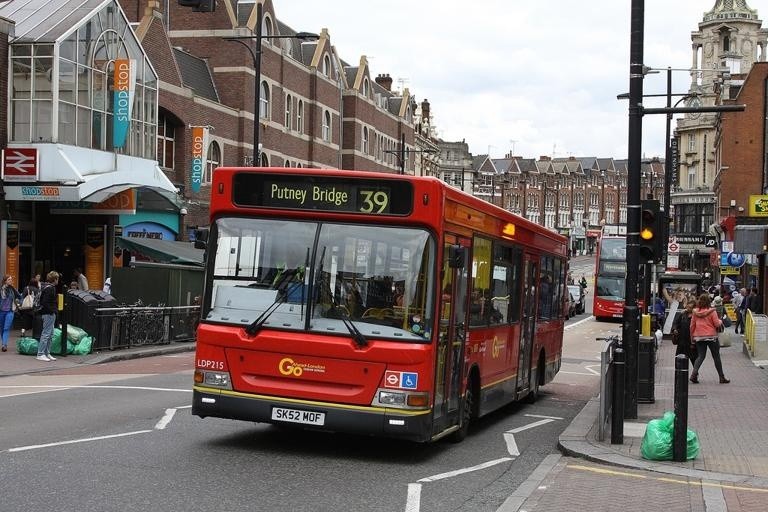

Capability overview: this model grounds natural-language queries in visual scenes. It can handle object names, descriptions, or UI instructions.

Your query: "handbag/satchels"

[11,301,20,313]
[672,329,680,344]
[718,333,732,346]
[22,295,35,309]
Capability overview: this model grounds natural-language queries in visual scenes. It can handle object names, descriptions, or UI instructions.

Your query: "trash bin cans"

[638,336,655,404]
[66,288,118,352]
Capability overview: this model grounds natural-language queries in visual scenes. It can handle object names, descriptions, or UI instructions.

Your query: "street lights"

[382,133,440,176]
[613,65,706,274]
[221,1,320,169]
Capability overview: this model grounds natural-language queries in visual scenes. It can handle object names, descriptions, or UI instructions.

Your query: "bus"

[189,164,571,449]
[590,221,655,324]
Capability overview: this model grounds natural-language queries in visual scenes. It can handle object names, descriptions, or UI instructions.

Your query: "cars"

[566,284,586,322]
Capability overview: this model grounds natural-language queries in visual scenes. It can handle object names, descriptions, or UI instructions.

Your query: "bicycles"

[117,298,168,348]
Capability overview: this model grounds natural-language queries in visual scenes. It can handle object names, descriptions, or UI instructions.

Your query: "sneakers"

[720,377,730,383]
[690,374,699,383]
[37,353,56,362]
[2,345,7,351]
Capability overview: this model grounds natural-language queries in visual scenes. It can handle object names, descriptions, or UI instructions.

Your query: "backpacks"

[33,284,50,311]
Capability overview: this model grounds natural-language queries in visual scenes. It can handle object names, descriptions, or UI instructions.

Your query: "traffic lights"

[636,199,661,266]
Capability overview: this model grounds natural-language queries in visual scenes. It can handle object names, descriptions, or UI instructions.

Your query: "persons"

[568,246,593,258]
[262,243,305,302]
[578,277,589,294]
[673,288,762,383]
[0,267,89,361]
